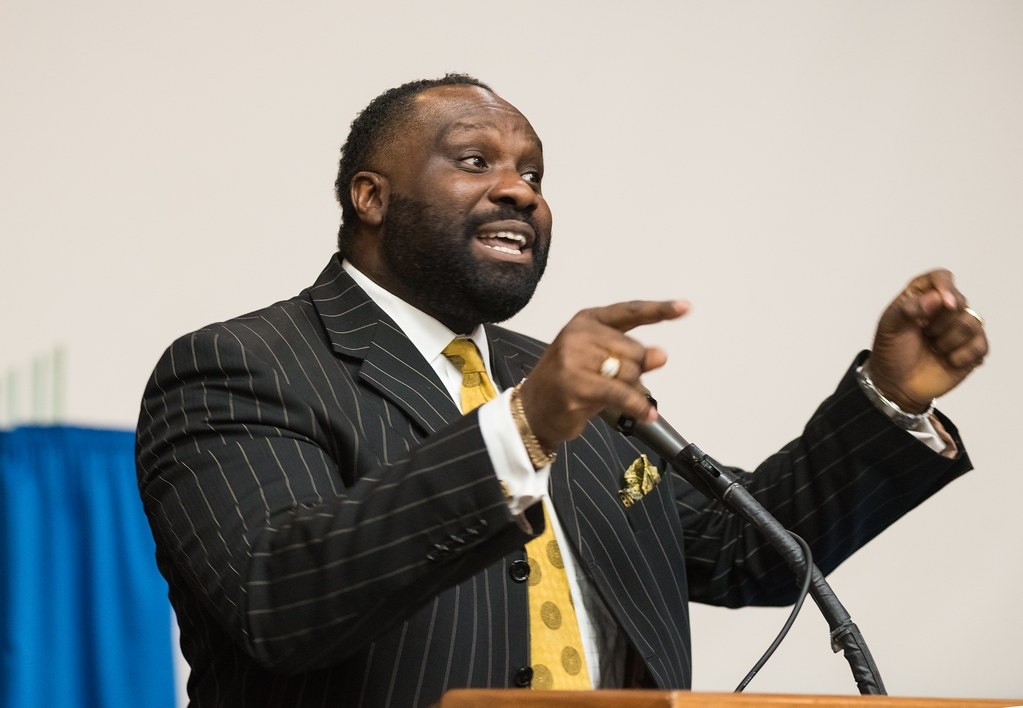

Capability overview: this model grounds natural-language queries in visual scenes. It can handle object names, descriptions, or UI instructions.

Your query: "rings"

[964,307,985,323]
[599,356,620,380]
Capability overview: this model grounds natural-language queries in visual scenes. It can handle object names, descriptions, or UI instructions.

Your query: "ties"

[442,337,592,690]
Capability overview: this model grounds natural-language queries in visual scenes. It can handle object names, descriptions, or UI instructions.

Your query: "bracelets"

[856,366,935,431]
[510,378,558,470]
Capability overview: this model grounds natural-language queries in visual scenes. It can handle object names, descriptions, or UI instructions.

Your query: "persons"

[135,74,989,708]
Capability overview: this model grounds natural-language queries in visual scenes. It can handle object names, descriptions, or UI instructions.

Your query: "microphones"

[597,385,738,501]
